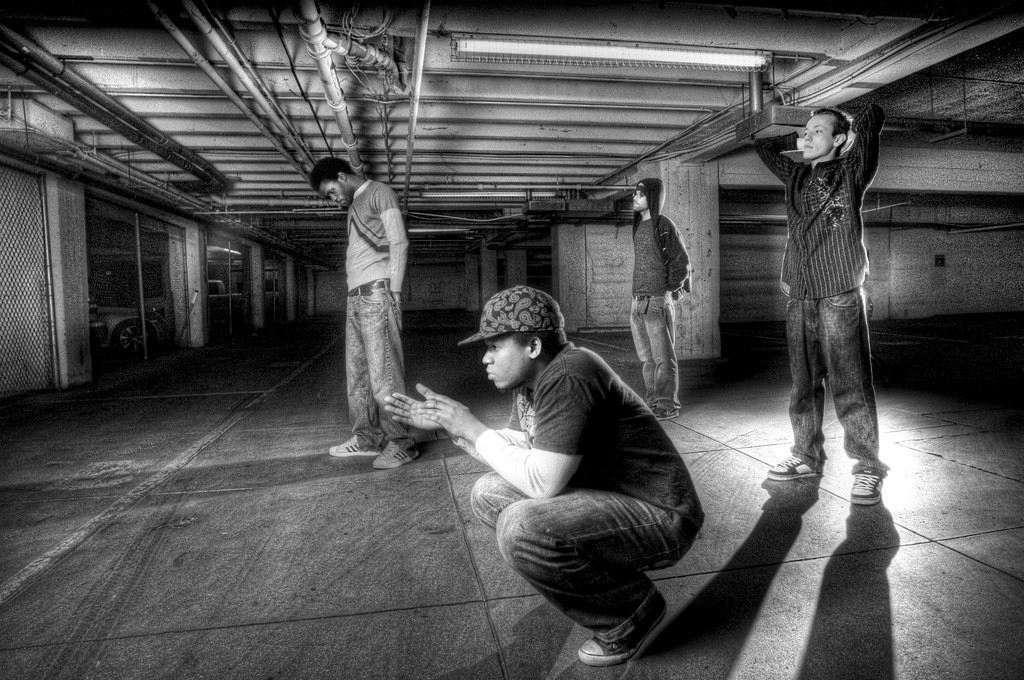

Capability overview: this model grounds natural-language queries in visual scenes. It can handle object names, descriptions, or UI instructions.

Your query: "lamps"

[449,31,774,73]
[419,189,557,200]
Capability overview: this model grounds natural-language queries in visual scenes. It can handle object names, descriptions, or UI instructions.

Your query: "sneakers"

[329,434,383,457]
[578,588,667,666]
[851,469,883,505]
[373,441,419,469]
[767,454,825,480]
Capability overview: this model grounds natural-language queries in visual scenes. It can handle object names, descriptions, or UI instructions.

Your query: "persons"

[310,157,420,469]
[385,286,705,666]
[754,103,891,506]
[629,177,693,420]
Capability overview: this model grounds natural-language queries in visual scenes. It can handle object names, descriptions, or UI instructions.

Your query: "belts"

[347,282,390,297]
[633,296,663,301]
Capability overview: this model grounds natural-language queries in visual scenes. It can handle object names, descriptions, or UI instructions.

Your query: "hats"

[634,182,648,196]
[457,286,565,347]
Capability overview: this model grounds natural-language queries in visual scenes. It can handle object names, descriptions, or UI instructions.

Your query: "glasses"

[632,193,646,198]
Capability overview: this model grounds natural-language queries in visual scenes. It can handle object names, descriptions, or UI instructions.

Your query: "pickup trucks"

[85,279,280,356]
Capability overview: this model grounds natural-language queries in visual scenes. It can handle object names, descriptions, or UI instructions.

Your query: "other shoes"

[650,405,679,421]
